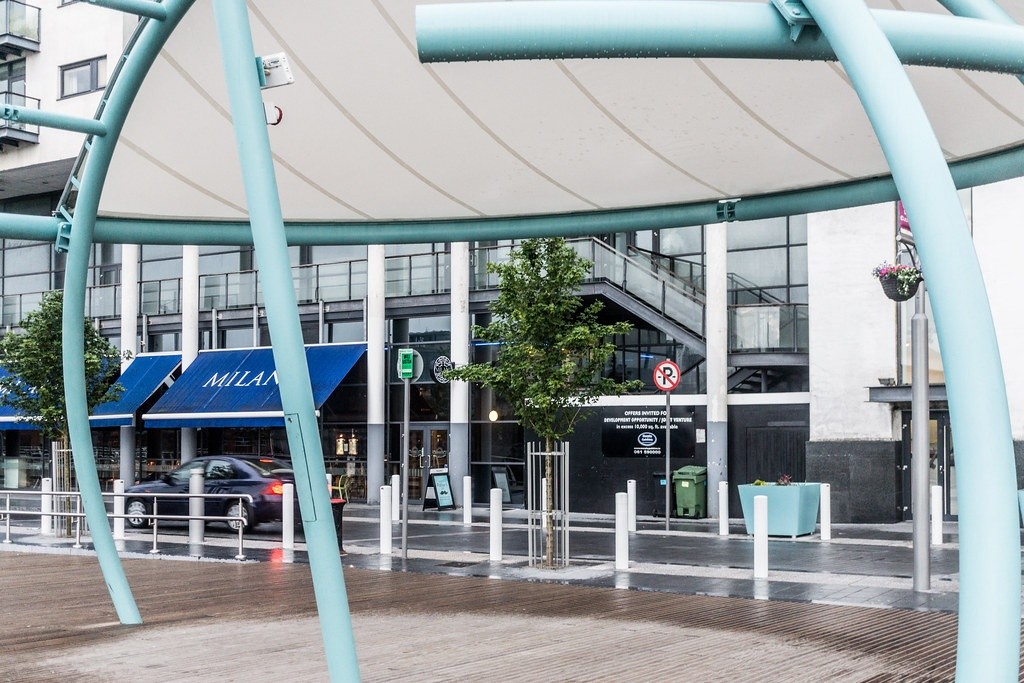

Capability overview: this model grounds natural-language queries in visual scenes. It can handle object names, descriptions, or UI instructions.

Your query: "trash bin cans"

[672,465,707,519]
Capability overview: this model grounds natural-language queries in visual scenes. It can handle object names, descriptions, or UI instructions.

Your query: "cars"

[124,454,299,533]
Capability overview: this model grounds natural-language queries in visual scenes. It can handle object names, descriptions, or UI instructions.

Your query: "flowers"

[873,263,919,295]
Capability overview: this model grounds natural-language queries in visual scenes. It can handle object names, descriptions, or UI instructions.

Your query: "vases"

[879,275,924,302]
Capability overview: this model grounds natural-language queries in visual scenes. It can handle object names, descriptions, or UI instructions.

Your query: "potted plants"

[737,474,821,538]
